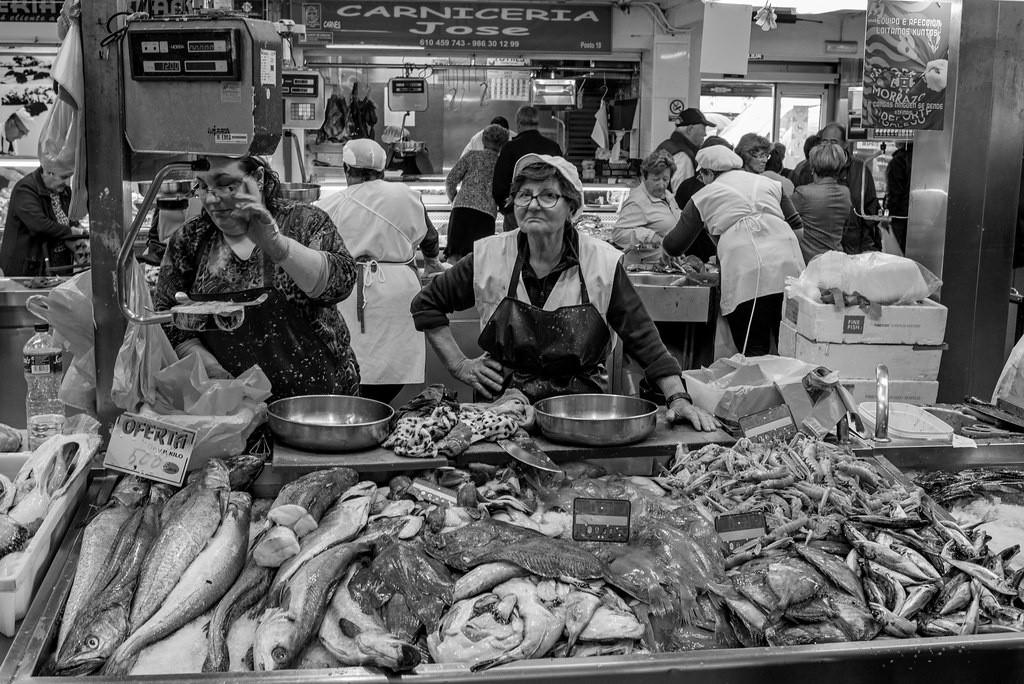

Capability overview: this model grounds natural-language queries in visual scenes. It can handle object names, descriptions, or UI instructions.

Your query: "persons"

[154,153,364,398]
[380,125,435,175]
[491,106,563,232]
[0,160,90,277]
[411,153,722,432]
[310,138,447,411]
[442,124,509,263]
[865,0,949,131]
[460,116,519,158]
[610,107,912,356]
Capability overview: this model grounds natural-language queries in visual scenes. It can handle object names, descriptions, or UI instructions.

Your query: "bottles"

[22,320,70,452]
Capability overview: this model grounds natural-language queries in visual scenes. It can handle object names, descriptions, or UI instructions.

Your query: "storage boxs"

[142,400,255,473]
[776,281,949,409]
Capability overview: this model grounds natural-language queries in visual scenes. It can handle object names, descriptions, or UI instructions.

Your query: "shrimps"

[658,432,890,546]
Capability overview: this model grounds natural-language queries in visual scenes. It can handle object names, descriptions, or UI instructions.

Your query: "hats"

[512,153,586,223]
[342,139,387,171]
[675,108,717,127]
[695,145,743,172]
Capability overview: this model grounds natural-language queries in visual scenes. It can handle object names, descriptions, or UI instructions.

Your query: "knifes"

[496,436,563,474]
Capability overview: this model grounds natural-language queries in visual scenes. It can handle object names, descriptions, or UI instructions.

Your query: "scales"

[847,86,909,224]
[388,76,429,157]
[281,70,324,203]
[103,9,283,326]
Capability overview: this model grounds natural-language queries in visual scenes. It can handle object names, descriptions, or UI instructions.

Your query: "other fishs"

[724,511,1024,647]
[38,454,726,676]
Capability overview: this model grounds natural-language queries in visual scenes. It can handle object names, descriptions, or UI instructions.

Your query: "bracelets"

[667,392,693,410]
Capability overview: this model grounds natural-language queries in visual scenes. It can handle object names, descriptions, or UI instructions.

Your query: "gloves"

[420,255,445,279]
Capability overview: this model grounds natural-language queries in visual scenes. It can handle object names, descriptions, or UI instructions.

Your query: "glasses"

[514,191,564,209]
[754,153,772,161]
[191,171,254,199]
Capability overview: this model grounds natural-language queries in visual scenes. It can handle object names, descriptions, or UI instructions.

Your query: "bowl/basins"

[138,180,191,198]
[277,181,320,206]
[532,393,659,446]
[266,394,394,451]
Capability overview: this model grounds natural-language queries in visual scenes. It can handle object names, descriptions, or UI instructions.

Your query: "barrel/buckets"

[858,400,954,442]
